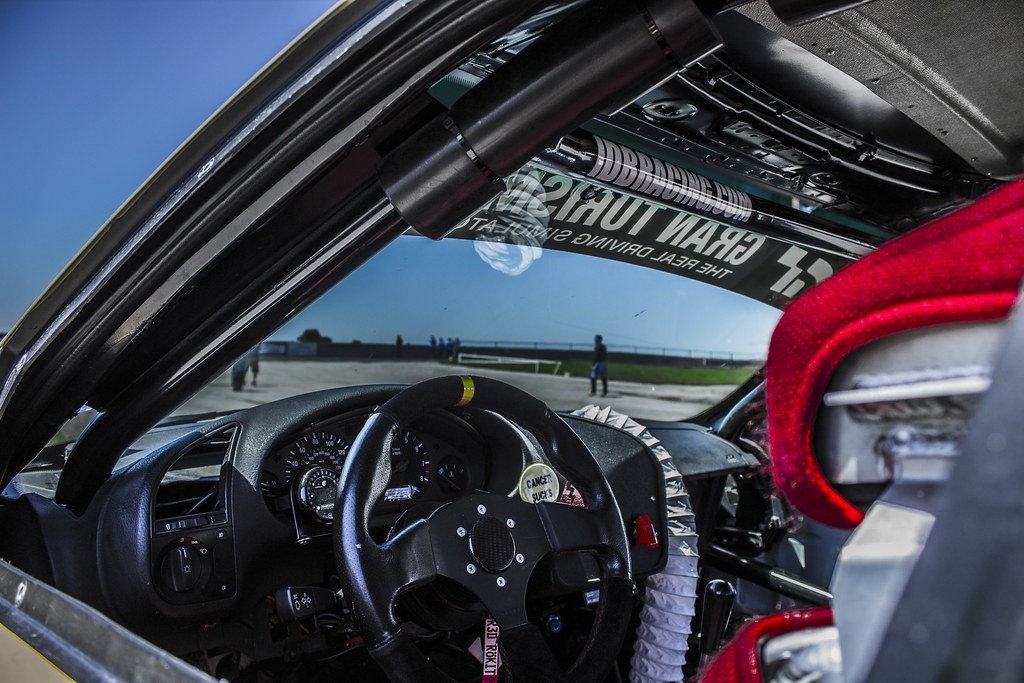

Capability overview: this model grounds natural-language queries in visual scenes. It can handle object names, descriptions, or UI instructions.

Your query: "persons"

[232,344,261,389]
[431,336,437,357]
[590,335,608,397]
[396,335,402,357]
[453,338,459,359]
[446,337,453,358]
[438,338,444,357]
